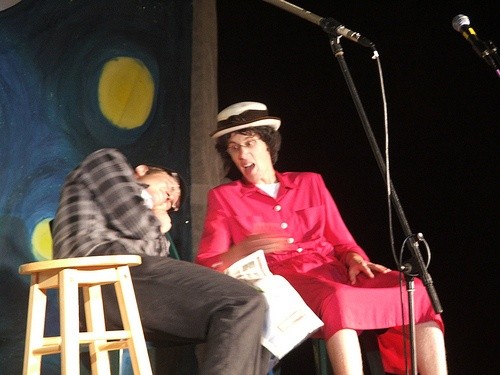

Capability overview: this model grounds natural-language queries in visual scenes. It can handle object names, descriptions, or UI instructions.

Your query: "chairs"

[312,332,384,375]
[49,219,198,375]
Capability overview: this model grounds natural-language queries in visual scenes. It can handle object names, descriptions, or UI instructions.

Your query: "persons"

[196,100,449,375]
[50,147,275,375]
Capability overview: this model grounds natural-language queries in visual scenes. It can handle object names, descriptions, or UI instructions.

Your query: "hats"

[210,102,281,138]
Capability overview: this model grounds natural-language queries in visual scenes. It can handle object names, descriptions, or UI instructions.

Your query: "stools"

[18,254,154,375]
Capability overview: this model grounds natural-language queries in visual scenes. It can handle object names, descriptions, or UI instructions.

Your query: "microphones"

[452,14,498,72]
[263,0,376,50]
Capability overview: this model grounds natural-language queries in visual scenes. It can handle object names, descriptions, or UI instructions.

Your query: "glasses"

[225,137,261,154]
[150,169,180,211]
